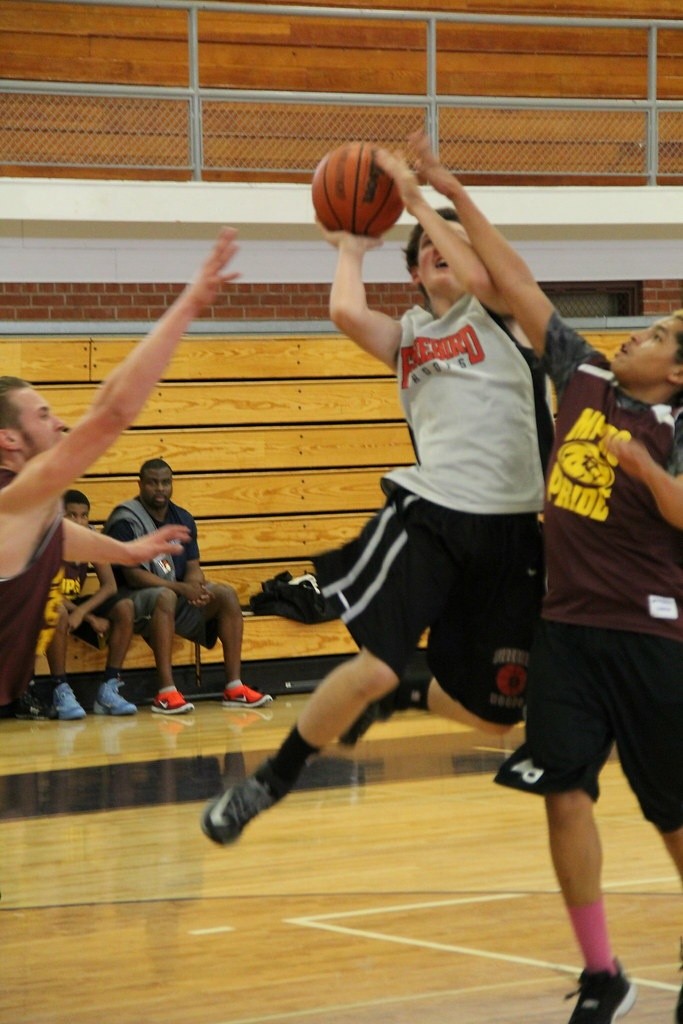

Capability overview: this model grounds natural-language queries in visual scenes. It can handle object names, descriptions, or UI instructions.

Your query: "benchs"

[0,611,429,708]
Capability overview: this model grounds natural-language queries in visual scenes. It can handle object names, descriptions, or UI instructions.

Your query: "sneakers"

[94,678,137,715]
[15,692,49,720]
[49,682,86,720]
[151,690,195,714]
[564,957,637,1024]
[339,667,407,747]
[676,937,683,1024]
[223,683,273,707]
[199,772,276,845]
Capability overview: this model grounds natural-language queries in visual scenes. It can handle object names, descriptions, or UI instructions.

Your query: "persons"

[0,226,241,719]
[100,457,276,713]
[49,487,140,716]
[199,125,573,845]
[403,124,683,1023]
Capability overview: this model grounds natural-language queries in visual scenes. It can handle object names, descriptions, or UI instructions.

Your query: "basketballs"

[312,142,404,236]
